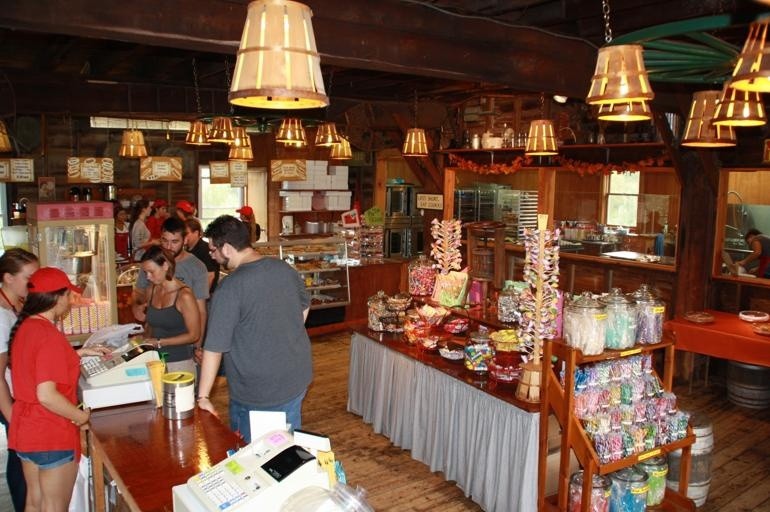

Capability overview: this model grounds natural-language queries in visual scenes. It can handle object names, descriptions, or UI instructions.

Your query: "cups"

[281,216,293,235]
[305,221,333,234]
[503,132,529,147]
[13,212,20,219]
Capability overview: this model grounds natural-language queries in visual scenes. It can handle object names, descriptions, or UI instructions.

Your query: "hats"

[153,199,167,207]
[176,199,193,214]
[236,206,252,216]
[27,268,84,293]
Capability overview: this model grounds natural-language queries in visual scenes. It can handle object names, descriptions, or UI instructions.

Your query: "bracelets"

[197,396,209,401]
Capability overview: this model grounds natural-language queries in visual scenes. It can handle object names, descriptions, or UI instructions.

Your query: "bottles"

[294,220,302,234]
[366,252,670,512]
[429,127,480,150]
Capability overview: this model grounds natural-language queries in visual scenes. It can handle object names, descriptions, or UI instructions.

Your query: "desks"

[346,295,562,512]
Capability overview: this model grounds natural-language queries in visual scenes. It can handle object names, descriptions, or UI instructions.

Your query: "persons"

[240,206,260,245]
[0,247,40,512]
[644,211,664,234]
[114,200,220,397]
[196,215,314,445]
[734,229,769,281]
[7,265,90,512]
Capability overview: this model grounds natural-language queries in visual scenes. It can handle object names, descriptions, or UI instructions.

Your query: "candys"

[608,468,648,512]
[635,302,667,347]
[557,352,690,466]
[566,469,608,511]
[518,225,560,341]
[559,306,606,357]
[428,215,464,276]
[402,316,428,346]
[463,332,497,375]
[496,294,520,324]
[629,455,669,508]
[406,261,438,297]
[603,307,638,351]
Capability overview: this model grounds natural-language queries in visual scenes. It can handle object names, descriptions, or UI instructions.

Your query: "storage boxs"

[280,161,352,211]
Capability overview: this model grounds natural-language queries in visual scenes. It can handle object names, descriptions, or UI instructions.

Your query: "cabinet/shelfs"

[443,166,556,240]
[375,155,407,213]
[88,398,248,511]
[64,188,158,221]
[248,162,419,337]
[538,327,697,511]
